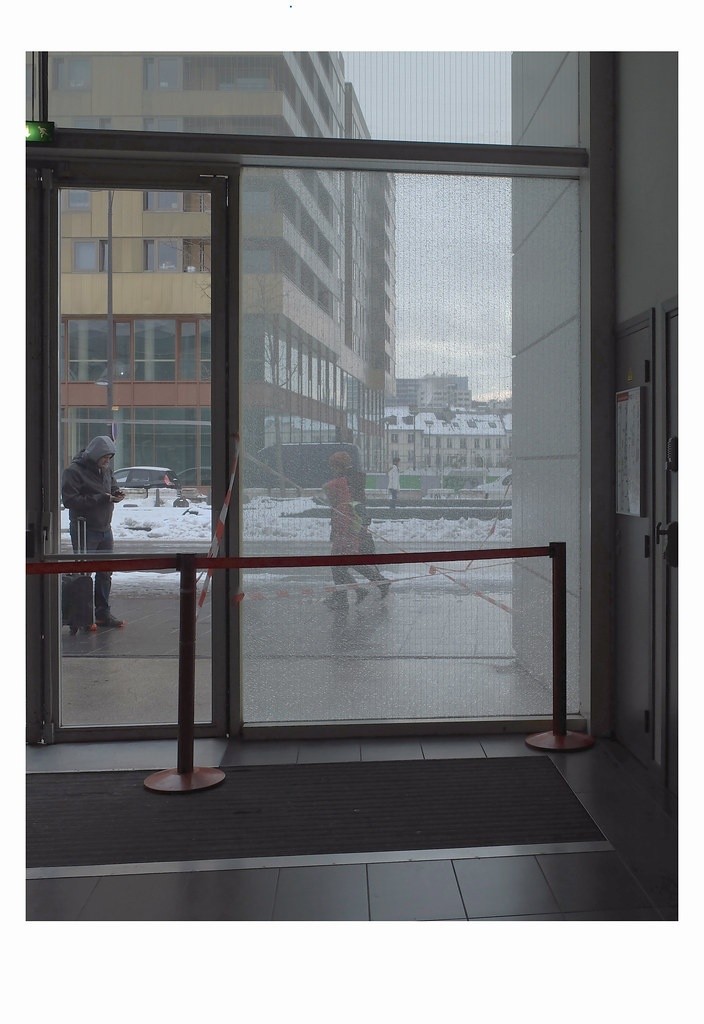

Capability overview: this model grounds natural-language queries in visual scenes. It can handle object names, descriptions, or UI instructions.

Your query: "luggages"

[62,517,93,628]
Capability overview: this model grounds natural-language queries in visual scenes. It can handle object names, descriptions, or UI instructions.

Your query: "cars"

[470,465,514,491]
[172,468,226,486]
[108,466,180,490]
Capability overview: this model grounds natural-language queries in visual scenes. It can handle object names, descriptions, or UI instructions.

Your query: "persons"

[436,452,443,475]
[388,458,401,509]
[62,436,126,632]
[324,451,391,603]
[322,478,370,611]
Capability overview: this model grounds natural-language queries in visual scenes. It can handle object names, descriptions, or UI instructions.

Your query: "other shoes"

[327,584,348,610]
[355,586,369,605]
[96,613,123,626]
[379,578,391,598]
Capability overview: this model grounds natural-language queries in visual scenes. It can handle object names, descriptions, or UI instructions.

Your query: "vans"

[243,442,367,489]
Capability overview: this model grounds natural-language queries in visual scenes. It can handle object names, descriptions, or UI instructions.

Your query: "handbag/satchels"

[358,532,375,553]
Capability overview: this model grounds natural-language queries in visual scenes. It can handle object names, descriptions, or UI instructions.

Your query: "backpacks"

[337,502,362,533]
